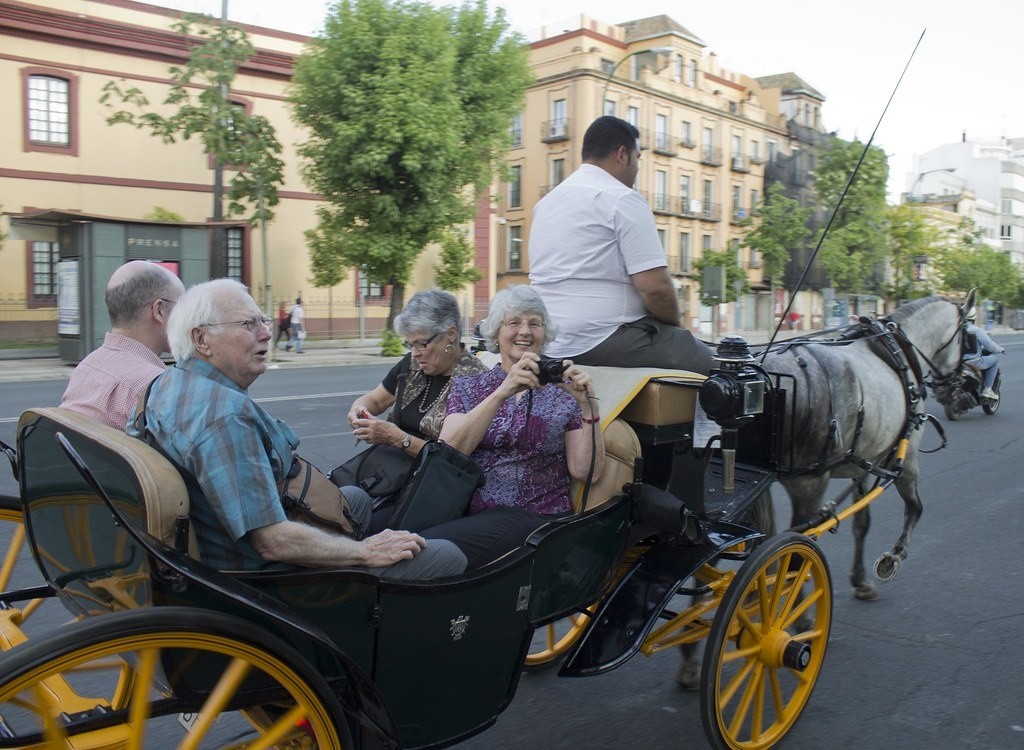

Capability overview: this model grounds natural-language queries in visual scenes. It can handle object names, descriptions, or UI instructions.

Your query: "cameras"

[529,359,565,383]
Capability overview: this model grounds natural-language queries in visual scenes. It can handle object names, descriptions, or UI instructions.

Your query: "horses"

[678,287,981,691]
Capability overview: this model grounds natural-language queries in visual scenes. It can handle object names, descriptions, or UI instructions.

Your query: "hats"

[295,298,303,304]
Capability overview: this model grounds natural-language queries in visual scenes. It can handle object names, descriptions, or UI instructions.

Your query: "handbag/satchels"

[279,310,290,330]
[331,435,414,497]
[384,442,484,531]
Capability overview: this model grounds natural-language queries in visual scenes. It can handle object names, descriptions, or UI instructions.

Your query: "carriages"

[0,282,979,750]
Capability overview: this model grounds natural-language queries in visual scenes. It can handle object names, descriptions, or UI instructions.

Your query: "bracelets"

[581,413,599,424]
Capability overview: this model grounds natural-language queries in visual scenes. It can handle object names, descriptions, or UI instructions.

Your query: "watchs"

[400,432,412,451]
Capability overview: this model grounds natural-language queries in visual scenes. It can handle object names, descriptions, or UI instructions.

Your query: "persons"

[787,308,799,336]
[529,116,720,376]
[59,259,185,433]
[273,302,291,352]
[124,276,468,582]
[287,297,305,353]
[411,285,604,569]
[328,289,488,505]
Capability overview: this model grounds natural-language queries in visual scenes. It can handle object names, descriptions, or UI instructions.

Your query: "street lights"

[907,168,956,206]
[601,46,676,117]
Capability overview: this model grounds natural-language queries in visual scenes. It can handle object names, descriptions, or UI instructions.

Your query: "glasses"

[502,318,543,329]
[401,333,438,351]
[199,317,275,333]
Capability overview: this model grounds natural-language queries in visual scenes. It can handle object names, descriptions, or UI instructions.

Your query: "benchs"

[475,351,709,452]
[523,418,644,628]
[14,406,382,708]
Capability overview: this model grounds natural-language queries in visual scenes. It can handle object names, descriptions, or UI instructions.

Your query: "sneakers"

[982,387,999,400]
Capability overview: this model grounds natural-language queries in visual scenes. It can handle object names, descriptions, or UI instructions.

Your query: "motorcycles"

[933,366,1002,422]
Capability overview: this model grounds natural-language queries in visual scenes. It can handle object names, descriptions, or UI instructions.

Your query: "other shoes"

[271,346,304,353]
[283,453,360,545]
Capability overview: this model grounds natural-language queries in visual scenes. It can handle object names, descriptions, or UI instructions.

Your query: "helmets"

[960,305,976,320]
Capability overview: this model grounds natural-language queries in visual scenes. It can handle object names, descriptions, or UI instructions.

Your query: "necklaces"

[418,376,452,413]
